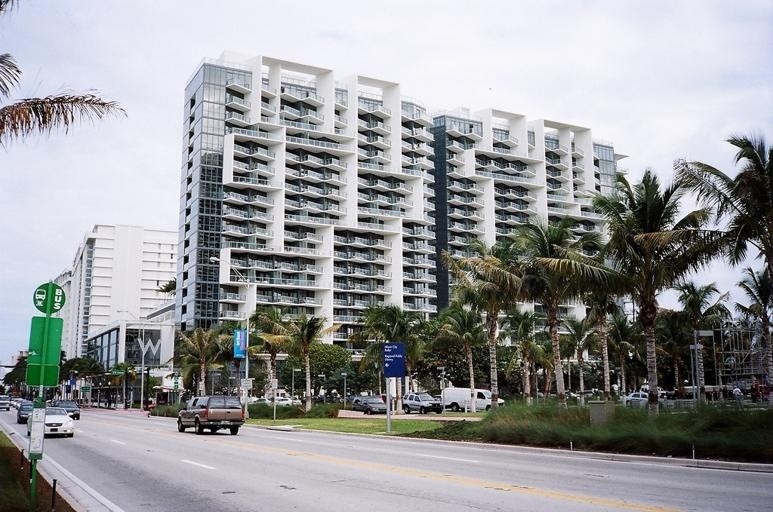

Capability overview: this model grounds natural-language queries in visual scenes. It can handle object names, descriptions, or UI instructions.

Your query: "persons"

[666,385,770,412]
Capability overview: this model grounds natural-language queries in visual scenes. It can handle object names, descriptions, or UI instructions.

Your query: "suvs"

[403,394,444,414]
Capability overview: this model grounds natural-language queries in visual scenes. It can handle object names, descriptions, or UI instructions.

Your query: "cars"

[0,393,82,440]
[575,385,674,409]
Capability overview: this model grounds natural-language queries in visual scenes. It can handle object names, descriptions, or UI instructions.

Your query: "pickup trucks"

[251,386,392,416]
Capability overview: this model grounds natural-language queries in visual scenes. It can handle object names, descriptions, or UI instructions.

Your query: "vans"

[177,394,246,435]
[442,388,505,412]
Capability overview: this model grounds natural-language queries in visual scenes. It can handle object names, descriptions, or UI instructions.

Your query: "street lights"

[209,256,249,419]
[116,309,146,413]
